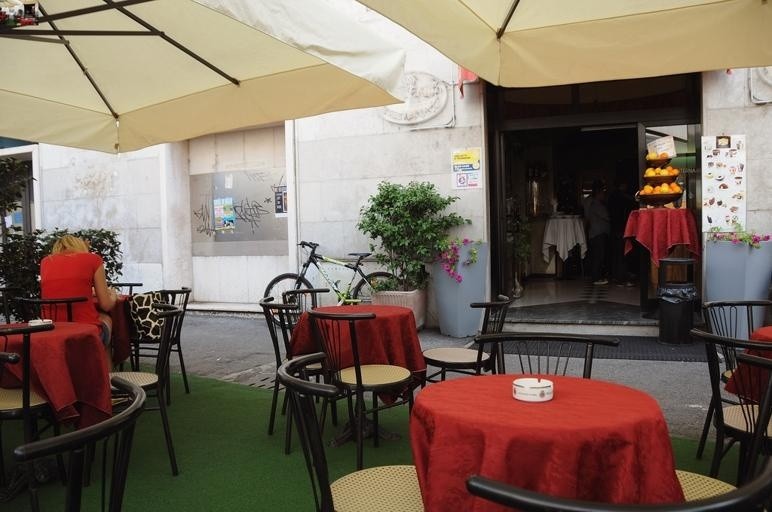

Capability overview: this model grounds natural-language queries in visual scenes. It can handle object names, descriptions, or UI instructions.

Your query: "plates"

[707,171,714,177]
[548,215,581,219]
[716,174,724,180]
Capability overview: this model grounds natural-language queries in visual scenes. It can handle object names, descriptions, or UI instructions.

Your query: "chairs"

[672,352,768,507]
[693,296,771,461]
[467,462,772,511]
[687,328,772,486]
[14,376,146,512]
[2,280,192,495]
[273,350,422,510]
[473,330,620,379]
[256,286,513,473]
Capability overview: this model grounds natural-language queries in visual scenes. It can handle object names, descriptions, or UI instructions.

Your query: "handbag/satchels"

[126,291,167,341]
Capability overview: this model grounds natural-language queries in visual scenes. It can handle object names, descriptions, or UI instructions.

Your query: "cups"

[730,148,737,156]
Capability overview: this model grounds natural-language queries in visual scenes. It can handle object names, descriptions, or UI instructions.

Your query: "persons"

[584,185,611,285]
[608,177,636,287]
[40,235,117,350]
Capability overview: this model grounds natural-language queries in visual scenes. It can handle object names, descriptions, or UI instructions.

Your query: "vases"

[368,284,428,332]
[706,240,771,347]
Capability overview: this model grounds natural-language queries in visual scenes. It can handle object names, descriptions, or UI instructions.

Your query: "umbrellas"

[0,0,404,154]
[358,2,772,89]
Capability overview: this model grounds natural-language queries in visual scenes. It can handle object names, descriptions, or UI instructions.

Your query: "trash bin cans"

[656,258,697,347]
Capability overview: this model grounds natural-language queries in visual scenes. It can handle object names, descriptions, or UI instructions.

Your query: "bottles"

[735,177,743,185]
[726,215,730,224]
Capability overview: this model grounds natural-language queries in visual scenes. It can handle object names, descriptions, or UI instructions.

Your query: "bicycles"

[264,241,402,330]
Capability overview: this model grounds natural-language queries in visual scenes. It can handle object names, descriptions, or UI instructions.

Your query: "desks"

[410,370,691,510]
[720,325,770,484]
[540,217,588,278]
[628,208,696,319]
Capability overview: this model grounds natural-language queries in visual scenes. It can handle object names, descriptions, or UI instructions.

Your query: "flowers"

[708,226,770,248]
[352,178,482,288]
